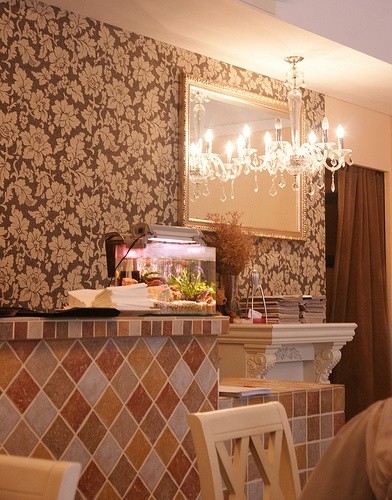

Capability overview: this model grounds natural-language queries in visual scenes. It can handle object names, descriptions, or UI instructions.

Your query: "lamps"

[188,84,293,203]
[244,56,352,197]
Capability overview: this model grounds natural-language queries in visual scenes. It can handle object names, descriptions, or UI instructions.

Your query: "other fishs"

[145,258,151,268]
[180,259,187,267]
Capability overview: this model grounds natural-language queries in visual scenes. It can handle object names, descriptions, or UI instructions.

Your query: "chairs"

[188,400,302,500]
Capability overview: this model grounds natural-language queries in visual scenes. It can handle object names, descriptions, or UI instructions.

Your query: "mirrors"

[178,74,308,241]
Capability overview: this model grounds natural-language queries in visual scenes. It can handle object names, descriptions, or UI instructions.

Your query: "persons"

[297,397,392,500]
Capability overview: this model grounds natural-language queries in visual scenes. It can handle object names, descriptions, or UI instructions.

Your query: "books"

[219,386,272,398]
[240,296,326,324]
